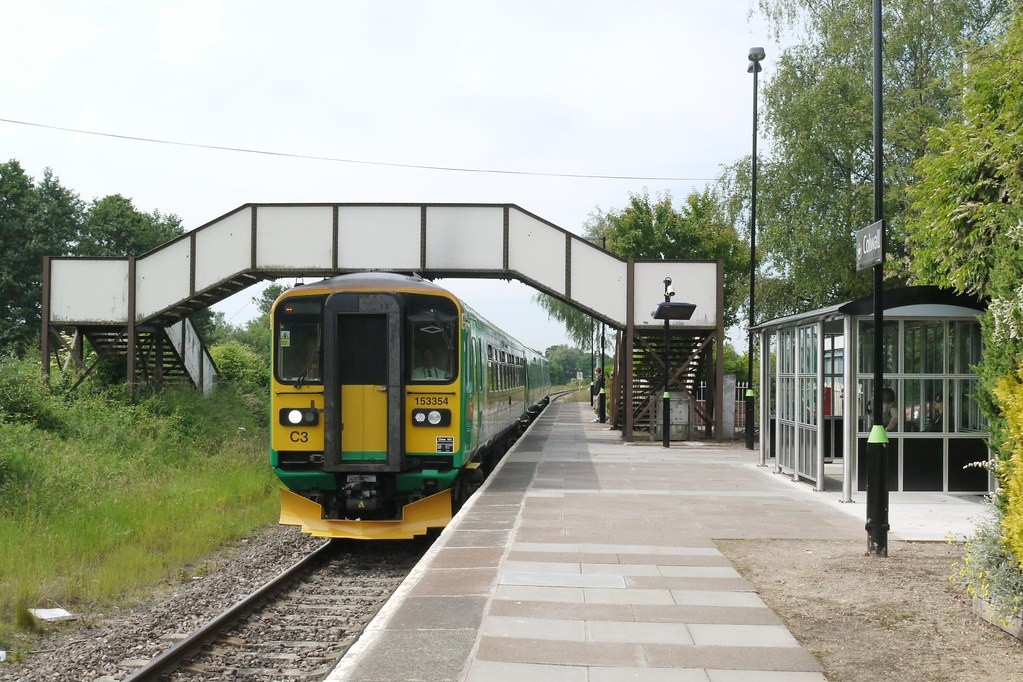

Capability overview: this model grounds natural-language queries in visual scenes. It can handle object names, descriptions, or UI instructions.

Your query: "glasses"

[934,400,942,403]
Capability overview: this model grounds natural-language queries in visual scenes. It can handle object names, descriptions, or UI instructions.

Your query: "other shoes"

[590,418,600,423]
[605,415,609,422]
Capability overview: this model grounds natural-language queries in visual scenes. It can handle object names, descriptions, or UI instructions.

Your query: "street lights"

[584,237,605,424]
[745,46,766,451]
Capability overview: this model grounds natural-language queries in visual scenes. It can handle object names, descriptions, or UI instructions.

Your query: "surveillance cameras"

[667,285,675,296]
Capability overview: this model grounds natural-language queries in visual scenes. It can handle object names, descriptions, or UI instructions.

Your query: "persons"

[882,388,898,432]
[412,344,450,380]
[590,368,609,423]
[914,392,969,432]
[304,360,321,380]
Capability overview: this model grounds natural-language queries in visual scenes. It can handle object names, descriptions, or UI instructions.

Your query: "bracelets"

[886,428,890,432]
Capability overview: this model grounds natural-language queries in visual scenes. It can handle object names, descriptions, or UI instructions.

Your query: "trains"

[269,274,552,539]
